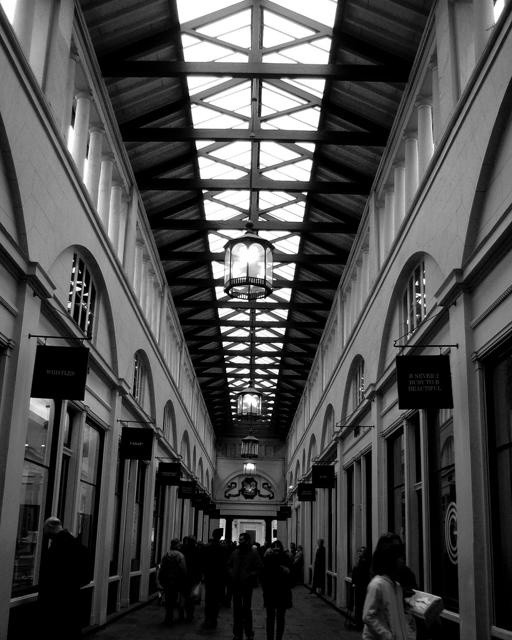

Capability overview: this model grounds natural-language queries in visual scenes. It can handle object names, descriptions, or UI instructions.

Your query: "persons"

[33,516,87,640]
[349,547,370,632]
[359,532,416,640]
[306,538,326,594]
[154,529,305,640]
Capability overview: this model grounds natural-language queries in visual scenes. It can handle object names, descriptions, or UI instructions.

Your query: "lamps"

[233,373,263,418]
[220,221,276,301]
[239,432,260,458]
